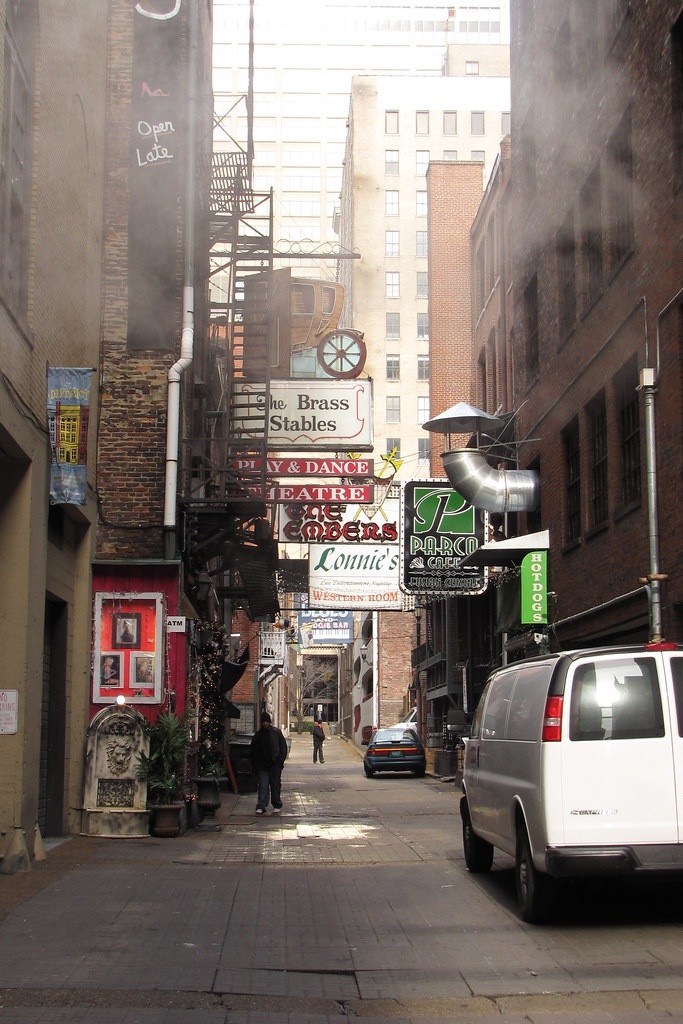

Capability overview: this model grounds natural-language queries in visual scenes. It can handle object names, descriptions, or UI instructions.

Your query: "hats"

[261,712,270,720]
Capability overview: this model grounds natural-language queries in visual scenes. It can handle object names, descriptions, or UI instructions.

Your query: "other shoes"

[273,808,280,812]
[321,761,325,764]
[256,808,266,813]
[314,762,315,763]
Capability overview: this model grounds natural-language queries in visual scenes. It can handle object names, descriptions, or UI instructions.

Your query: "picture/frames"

[112,613,141,649]
[100,651,124,689]
[129,651,155,688]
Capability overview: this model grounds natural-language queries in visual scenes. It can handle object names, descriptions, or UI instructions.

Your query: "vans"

[444,645,683,924]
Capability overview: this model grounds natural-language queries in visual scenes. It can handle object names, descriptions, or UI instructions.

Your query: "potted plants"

[133,712,188,837]
[191,752,228,832]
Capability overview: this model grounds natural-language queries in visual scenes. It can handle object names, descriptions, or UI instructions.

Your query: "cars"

[360,727,426,779]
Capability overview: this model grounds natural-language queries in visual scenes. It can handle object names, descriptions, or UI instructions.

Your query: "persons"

[102,656,120,686]
[246,711,287,816]
[313,719,325,764]
